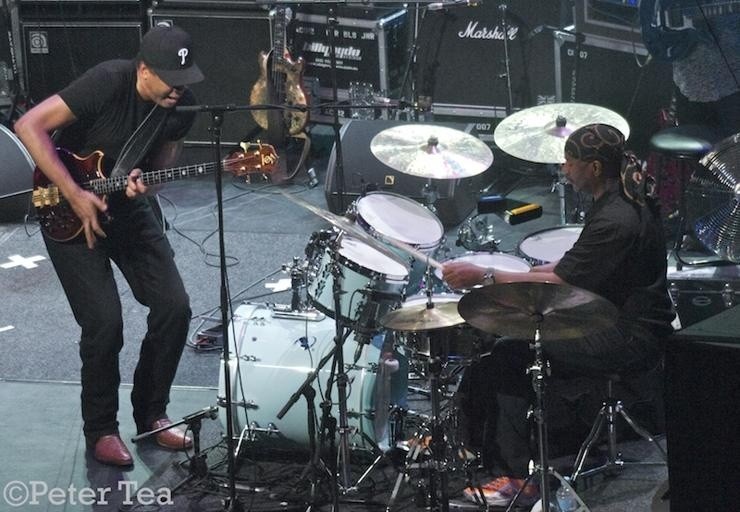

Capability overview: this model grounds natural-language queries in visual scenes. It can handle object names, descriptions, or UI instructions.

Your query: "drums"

[425,249,533,291]
[410,291,465,301]
[217,299,408,454]
[346,190,446,298]
[306,233,408,333]
[517,223,586,266]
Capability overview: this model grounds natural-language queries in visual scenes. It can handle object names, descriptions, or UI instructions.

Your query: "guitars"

[249,2,310,134]
[638,0,739,62]
[31,140,281,242]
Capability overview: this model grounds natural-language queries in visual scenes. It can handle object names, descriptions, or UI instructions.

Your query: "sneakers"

[393,436,477,469]
[463,475,540,507]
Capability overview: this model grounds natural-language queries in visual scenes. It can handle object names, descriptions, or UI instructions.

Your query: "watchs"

[479,267,497,287]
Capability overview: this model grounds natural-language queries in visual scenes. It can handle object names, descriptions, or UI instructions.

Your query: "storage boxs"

[653,248,740,330]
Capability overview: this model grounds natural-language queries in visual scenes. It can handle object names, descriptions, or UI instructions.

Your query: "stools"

[650,125,716,261]
[561,368,668,490]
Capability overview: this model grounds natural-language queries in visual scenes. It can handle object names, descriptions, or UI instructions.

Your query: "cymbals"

[493,103,630,163]
[370,124,494,180]
[380,301,466,332]
[458,281,618,342]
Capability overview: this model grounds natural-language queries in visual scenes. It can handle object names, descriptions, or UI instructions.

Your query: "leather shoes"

[137,417,194,450]
[85,433,135,468]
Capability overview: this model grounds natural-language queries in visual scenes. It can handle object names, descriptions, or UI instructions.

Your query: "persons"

[12,23,207,469]
[394,123,668,508]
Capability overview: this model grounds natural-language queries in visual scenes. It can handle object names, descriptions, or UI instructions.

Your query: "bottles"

[556,475,578,512]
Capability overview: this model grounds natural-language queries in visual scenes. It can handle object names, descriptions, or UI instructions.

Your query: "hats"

[140,23,205,88]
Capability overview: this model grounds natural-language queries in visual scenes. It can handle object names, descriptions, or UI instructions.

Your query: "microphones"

[175,103,238,114]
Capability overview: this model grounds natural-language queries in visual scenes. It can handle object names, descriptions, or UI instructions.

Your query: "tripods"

[165,458,247,493]
[502,390,589,512]
